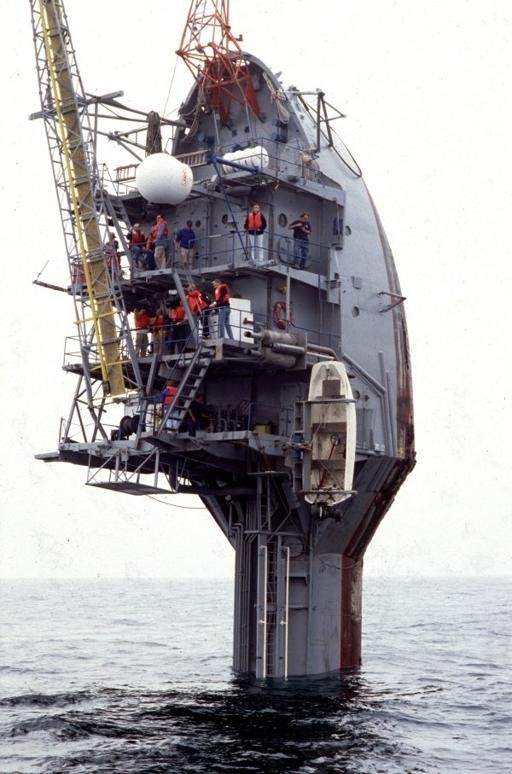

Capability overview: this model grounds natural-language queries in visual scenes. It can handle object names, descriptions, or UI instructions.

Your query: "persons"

[244,204,266,262]
[135,279,234,358]
[104,214,171,281]
[186,394,209,436]
[288,211,311,270]
[175,221,195,270]
[159,380,179,434]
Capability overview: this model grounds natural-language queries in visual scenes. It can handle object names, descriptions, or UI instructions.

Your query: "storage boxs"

[255,423,270,434]
